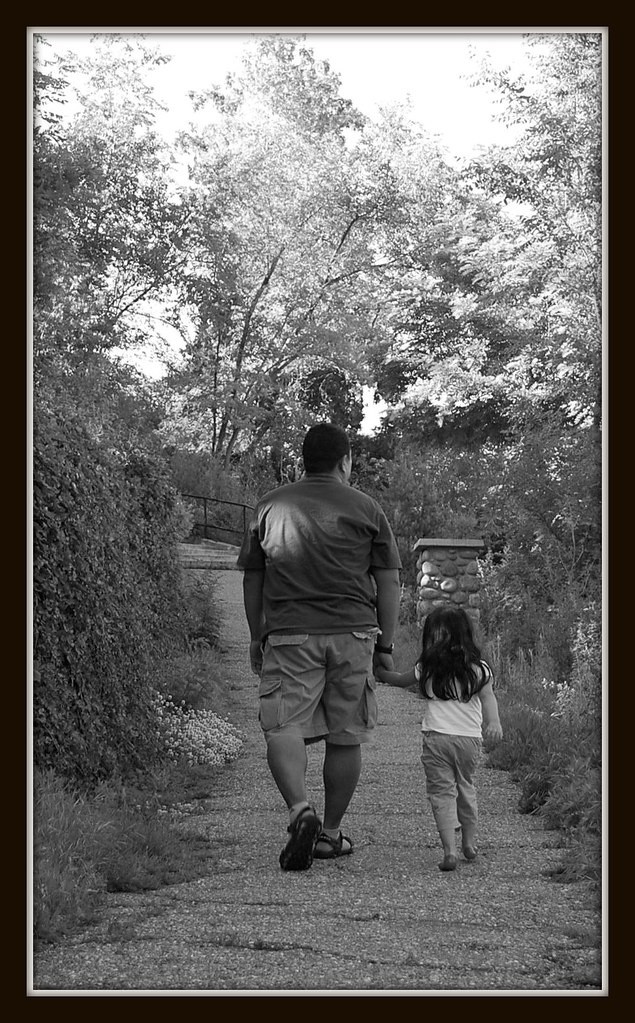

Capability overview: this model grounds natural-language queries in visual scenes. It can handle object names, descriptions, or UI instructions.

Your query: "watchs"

[375,643,394,654]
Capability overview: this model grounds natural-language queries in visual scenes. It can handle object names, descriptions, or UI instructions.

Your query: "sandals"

[278,806,322,871]
[313,831,353,859]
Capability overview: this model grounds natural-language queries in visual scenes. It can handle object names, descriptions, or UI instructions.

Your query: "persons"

[236,423,402,870]
[375,606,503,870]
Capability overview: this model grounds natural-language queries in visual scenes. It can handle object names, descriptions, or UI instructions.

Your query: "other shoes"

[463,846,478,859]
[438,854,458,870]
